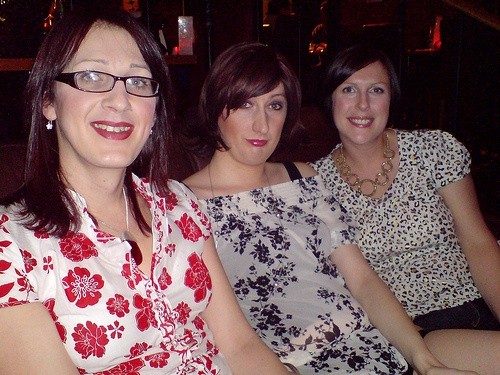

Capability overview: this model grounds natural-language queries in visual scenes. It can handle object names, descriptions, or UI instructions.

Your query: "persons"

[302,42,500,374]
[180,41,474,375]
[0,5,293,375]
[257,0,360,76]
[0,0,59,139]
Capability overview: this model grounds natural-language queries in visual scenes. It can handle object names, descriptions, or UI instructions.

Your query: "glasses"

[52,70,161,98]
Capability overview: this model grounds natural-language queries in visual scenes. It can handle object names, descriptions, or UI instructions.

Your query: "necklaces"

[92,186,139,249]
[336,130,395,197]
[206,159,289,287]
